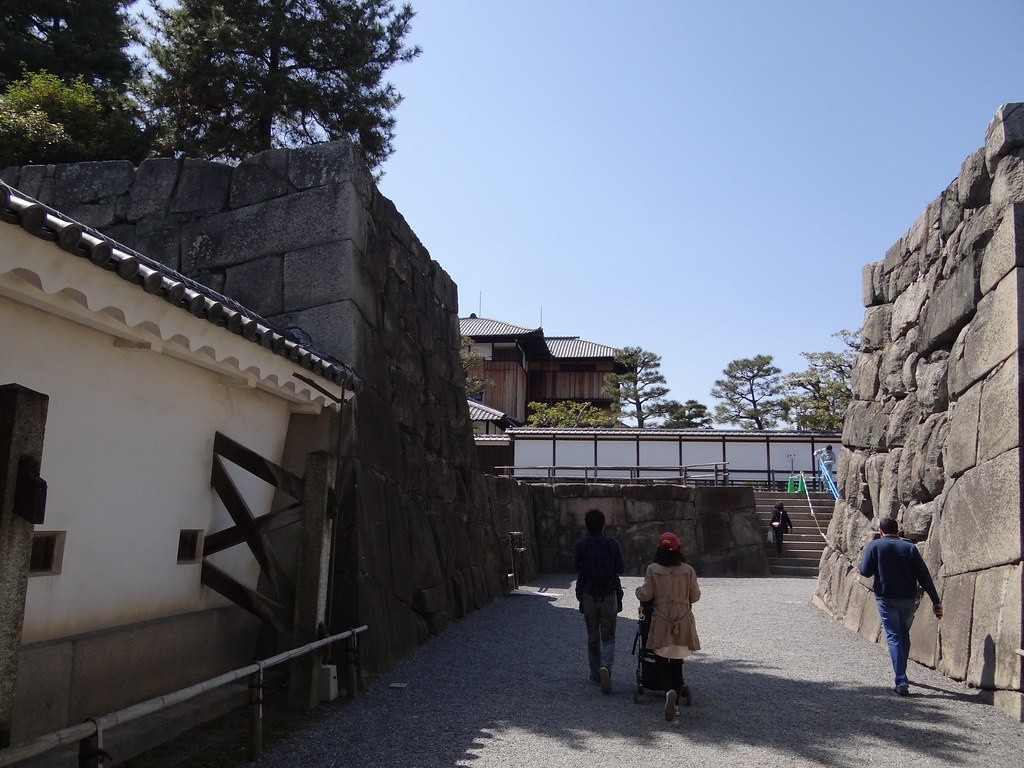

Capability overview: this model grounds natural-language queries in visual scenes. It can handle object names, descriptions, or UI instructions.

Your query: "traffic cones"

[797,475,805,492]
[787,477,795,493]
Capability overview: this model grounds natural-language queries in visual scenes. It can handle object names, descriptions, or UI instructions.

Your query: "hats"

[658,533,682,550]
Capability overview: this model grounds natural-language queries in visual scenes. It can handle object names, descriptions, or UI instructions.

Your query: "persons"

[814,444,836,493]
[573,508,625,694]
[769,501,793,555]
[635,533,702,722]
[859,518,943,695]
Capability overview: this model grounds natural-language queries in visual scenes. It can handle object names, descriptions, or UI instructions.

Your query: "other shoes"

[663,689,677,723]
[894,684,910,697]
[599,666,612,695]
[587,673,600,686]
[675,705,680,717]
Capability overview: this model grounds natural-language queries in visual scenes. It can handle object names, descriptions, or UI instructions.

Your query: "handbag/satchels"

[771,522,783,529]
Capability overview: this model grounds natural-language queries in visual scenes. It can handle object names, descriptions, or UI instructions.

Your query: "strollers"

[631,600,692,707]
